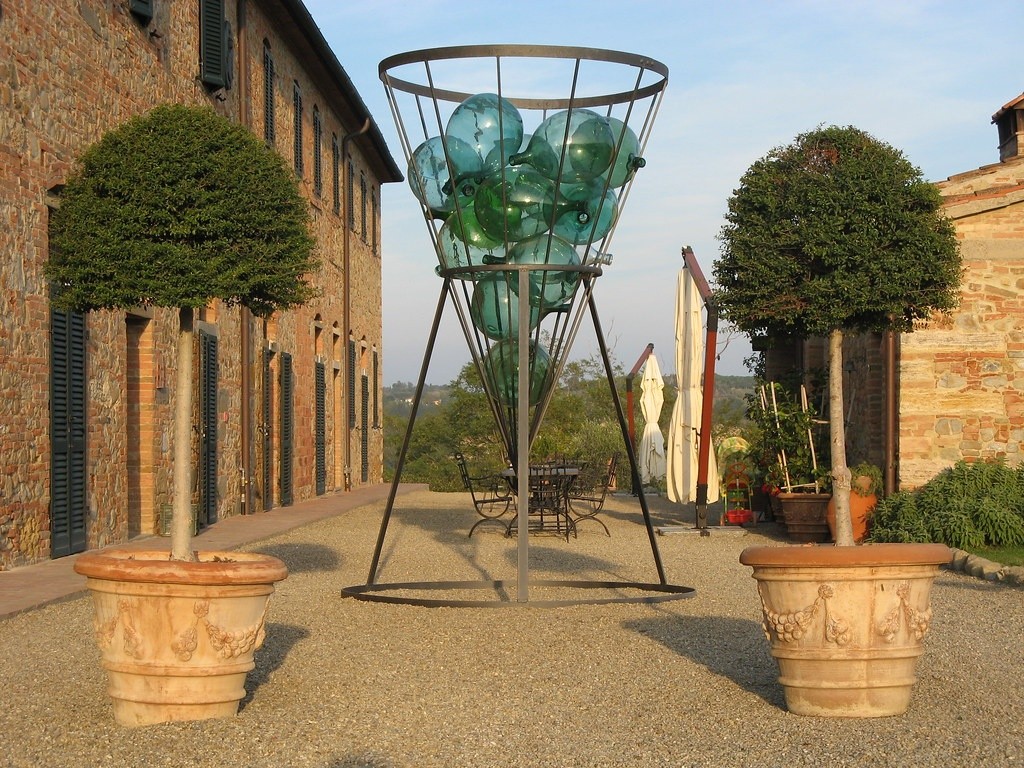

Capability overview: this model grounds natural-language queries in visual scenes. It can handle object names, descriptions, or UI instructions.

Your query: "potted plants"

[703,120,972,721]
[742,352,833,541]
[39,101,327,731]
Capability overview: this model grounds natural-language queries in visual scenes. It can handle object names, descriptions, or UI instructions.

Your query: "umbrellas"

[637,267,720,505]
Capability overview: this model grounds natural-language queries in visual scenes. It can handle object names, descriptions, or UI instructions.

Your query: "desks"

[501,466,579,537]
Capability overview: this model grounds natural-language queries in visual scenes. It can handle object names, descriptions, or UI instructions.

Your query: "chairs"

[454,450,623,544]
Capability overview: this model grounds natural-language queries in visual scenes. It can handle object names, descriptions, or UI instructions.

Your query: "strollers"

[716,436,758,529]
